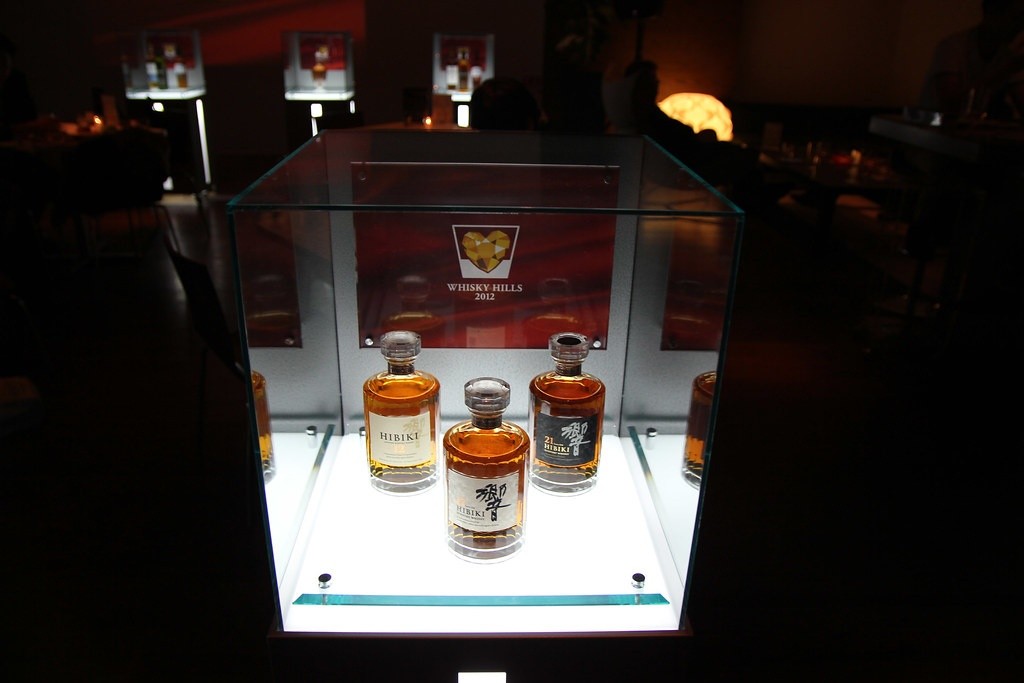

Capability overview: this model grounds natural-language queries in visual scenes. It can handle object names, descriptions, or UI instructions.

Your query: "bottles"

[530,334,604,495]
[249,366,279,484]
[682,371,717,484]
[444,375,528,563]
[360,331,441,494]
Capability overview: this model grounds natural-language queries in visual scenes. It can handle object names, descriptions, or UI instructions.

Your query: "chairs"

[603,83,733,191]
[166,242,245,460]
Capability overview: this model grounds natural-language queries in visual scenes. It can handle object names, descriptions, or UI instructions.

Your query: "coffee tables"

[731,131,925,249]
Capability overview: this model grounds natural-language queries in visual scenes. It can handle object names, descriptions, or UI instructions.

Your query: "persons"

[468,78,536,132]
[923,2,1024,124]
[0,35,38,144]
[618,60,690,148]
